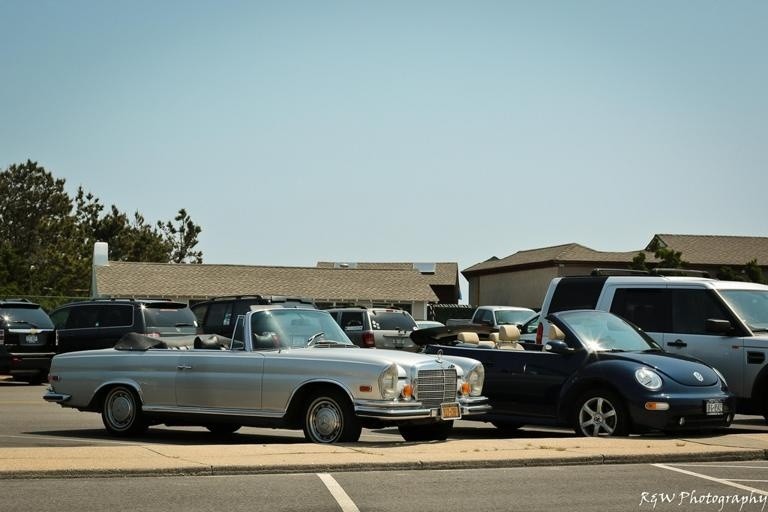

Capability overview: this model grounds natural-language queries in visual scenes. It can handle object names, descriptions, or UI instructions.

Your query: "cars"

[409,308,736,440]
[44,304,492,446]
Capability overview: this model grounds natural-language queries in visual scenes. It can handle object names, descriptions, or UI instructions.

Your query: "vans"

[535,267,768,422]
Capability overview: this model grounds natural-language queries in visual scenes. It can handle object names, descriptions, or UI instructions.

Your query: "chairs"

[455,323,564,351]
[251,332,277,351]
[194,335,221,349]
